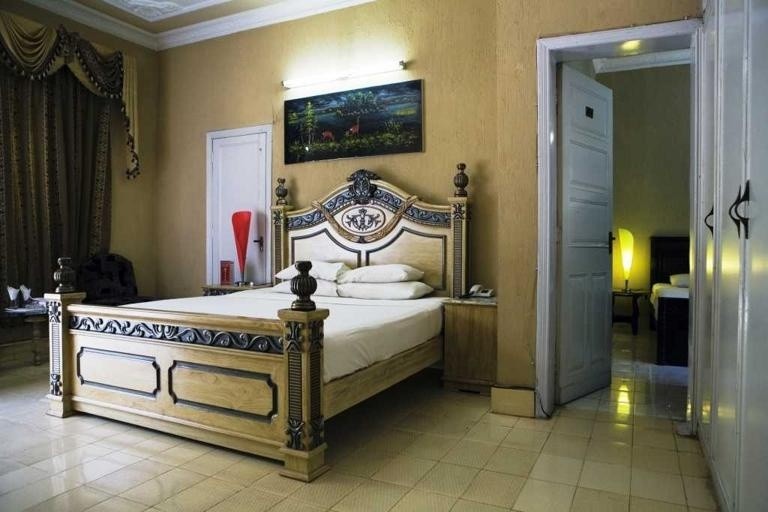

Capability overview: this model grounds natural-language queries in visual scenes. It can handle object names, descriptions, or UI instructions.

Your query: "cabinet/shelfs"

[685,0,767,512]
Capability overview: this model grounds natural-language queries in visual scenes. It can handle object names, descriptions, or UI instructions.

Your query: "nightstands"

[198,283,272,300]
[439,288,496,399]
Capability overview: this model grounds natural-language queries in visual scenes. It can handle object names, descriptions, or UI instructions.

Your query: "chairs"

[83,248,152,307]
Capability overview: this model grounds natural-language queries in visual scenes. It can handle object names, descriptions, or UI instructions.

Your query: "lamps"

[280,58,408,90]
[619,229,637,293]
[232,210,251,285]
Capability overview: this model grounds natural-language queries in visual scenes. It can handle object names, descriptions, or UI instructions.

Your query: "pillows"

[271,278,339,297]
[332,282,433,298]
[668,270,688,289]
[338,262,425,281]
[275,258,347,281]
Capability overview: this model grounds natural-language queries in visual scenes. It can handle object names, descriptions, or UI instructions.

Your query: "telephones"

[469,285,495,298]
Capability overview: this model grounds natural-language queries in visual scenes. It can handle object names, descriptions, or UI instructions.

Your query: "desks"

[4,308,49,370]
[608,288,647,336]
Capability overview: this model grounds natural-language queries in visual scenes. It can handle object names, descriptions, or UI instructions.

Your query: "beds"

[42,160,472,485]
[647,234,688,368]
